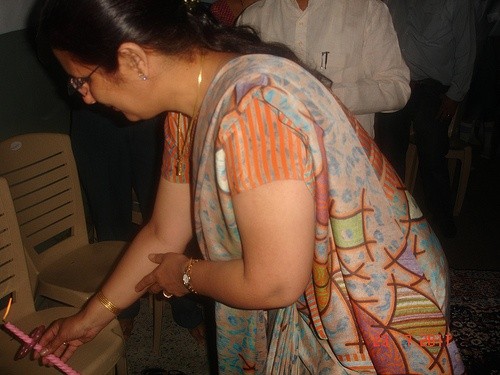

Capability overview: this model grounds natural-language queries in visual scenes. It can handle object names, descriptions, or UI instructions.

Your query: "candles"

[3,298,80,375]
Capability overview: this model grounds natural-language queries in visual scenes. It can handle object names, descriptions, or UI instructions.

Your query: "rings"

[163,291,173,299]
[63,343,69,346]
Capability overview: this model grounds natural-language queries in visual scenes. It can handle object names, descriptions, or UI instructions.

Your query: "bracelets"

[96,290,122,316]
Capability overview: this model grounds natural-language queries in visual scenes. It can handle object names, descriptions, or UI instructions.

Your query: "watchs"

[182,259,199,296]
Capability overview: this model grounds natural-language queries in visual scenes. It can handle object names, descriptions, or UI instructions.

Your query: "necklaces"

[178,55,205,175]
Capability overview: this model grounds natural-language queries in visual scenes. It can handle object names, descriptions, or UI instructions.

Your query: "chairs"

[0,177,127,375]
[0,132,163,355]
[404,112,472,217]
[73,99,150,227]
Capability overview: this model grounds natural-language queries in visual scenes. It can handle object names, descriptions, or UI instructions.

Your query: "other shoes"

[439,216,456,239]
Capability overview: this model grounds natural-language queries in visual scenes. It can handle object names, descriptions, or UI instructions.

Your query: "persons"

[210,0,262,27]
[377,0,477,236]
[67,78,203,328]
[236,0,411,141]
[34,0,467,375]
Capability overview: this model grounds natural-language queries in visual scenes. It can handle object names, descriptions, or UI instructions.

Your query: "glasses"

[70,60,107,96]
